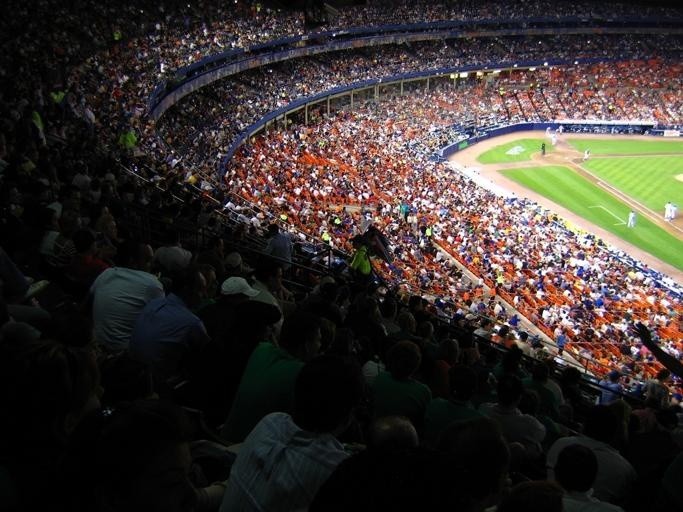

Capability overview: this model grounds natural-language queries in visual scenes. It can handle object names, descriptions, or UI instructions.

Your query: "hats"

[221,277,261,297]
[609,370,623,380]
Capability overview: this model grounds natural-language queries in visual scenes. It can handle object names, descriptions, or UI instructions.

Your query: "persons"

[0,2,683,512]
